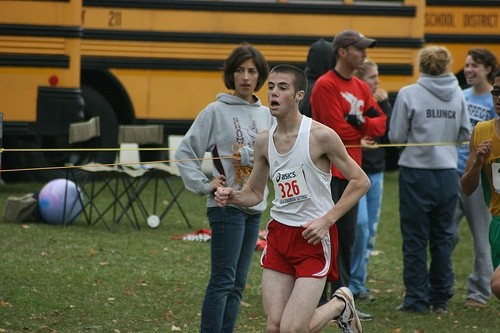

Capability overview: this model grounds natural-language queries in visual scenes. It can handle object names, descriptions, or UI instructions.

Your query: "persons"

[174,45,279,333]
[213,65,363,333]
[300,28,500,324]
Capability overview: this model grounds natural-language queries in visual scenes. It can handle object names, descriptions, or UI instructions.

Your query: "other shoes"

[464,298,484,310]
[353,309,374,322]
[396,303,412,312]
[432,305,447,313]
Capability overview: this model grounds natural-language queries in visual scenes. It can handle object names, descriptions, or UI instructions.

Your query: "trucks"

[0,0,500,185]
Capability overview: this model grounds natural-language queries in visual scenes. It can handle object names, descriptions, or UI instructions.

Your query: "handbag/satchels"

[3,193,40,224]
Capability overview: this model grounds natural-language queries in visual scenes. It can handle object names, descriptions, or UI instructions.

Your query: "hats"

[333,30,377,50]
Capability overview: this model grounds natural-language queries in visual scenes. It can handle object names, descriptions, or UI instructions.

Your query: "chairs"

[62,116,213,233]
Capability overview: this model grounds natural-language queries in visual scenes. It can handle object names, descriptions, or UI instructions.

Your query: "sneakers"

[332,286,363,333]
